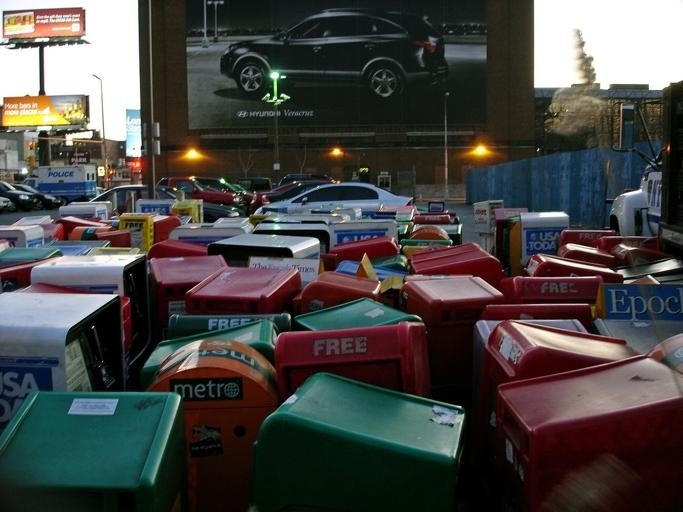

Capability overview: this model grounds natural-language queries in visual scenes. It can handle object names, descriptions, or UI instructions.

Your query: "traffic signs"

[70,150,89,164]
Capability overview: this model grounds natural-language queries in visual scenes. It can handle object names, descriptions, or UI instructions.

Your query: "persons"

[419,13,452,99]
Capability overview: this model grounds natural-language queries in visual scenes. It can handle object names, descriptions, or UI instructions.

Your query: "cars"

[13,184,56,210]
[250,183,419,219]
[0,197,11,213]
[158,169,338,212]
[58,183,242,227]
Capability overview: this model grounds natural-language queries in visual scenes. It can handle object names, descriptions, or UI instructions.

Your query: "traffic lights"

[132,159,141,170]
[28,141,35,150]
[108,169,115,176]
[26,155,35,165]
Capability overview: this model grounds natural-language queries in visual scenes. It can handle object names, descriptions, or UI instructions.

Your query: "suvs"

[0,181,33,212]
[219,9,450,100]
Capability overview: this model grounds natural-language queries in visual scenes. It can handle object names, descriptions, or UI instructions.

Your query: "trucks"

[609,81,683,262]
[22,165,97,206]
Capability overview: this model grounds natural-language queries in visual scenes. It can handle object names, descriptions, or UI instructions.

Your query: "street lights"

[92,73,106,167]
[119,143,123,178]
[206,0,223,42]
[1,35,91,168]
[261,69,291,179]
